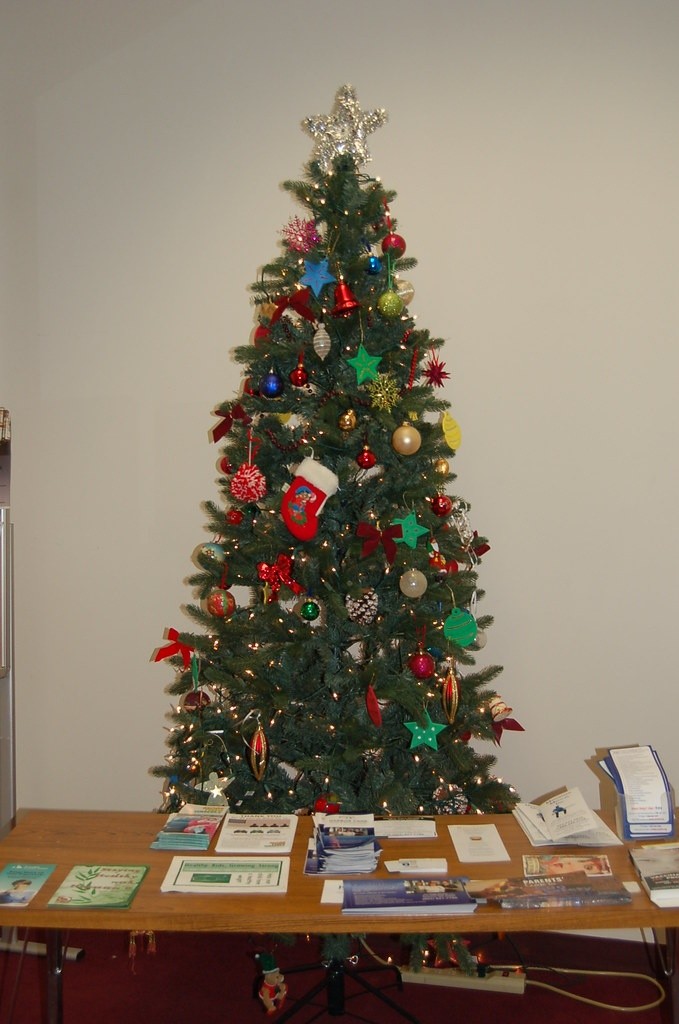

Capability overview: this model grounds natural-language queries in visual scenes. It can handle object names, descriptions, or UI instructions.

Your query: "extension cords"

[399,965,526,994]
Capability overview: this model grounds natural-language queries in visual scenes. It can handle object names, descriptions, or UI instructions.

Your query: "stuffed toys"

[255,953,288,1016]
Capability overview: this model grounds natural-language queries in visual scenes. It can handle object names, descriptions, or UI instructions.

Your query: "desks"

[0,807,679,1024]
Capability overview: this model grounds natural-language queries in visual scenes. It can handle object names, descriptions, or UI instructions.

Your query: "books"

[627,848,679,907]
[0,861,149,910]
[150,787,633,916]
